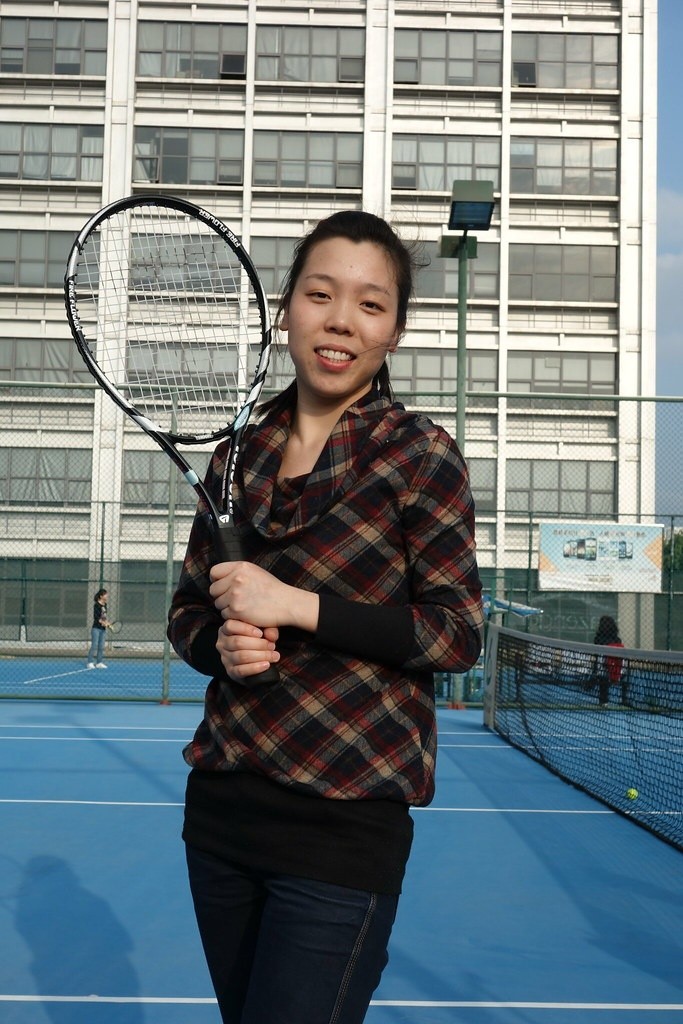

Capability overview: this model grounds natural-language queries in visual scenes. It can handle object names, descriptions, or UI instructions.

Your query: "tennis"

[627,788,638,799]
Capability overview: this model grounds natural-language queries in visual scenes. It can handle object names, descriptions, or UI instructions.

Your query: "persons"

[590,615,622,708]
[87,588,111,669]
[165,211,488,1024]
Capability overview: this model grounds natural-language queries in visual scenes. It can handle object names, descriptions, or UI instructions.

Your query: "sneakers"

[87,663,95,669]
[96,662,108,669]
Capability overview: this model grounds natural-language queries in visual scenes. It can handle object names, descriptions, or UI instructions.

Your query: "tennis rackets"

[107,620,123,634]
[62,194,283,692]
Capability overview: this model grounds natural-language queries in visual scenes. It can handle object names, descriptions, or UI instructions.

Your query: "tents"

[445,593,544,701]
